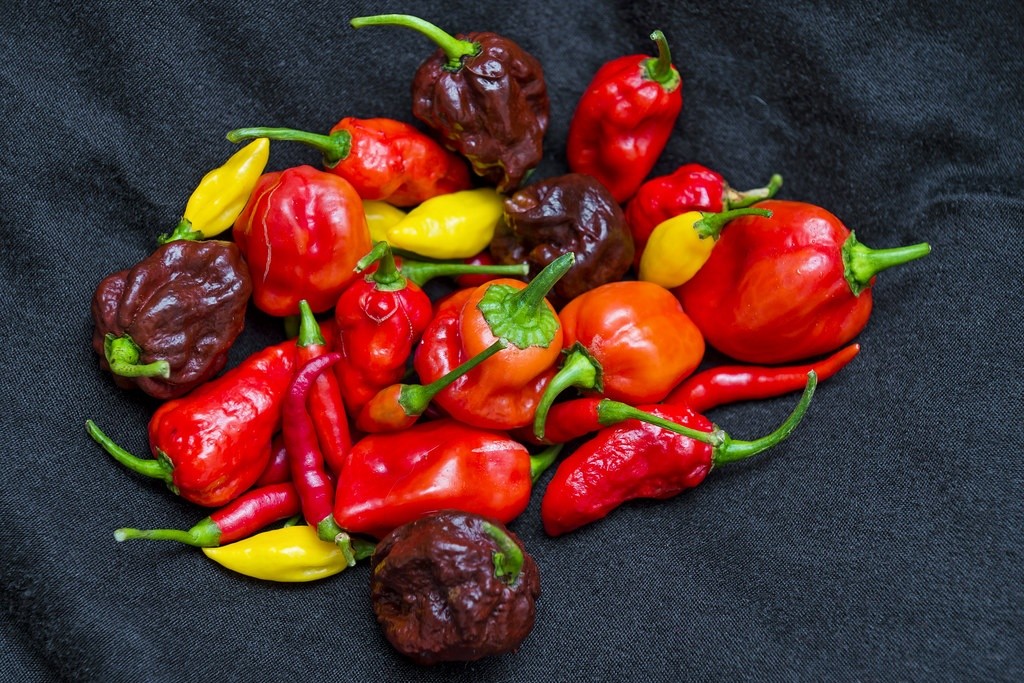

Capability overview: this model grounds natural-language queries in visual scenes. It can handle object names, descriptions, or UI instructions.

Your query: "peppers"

[84,14,931,661]
